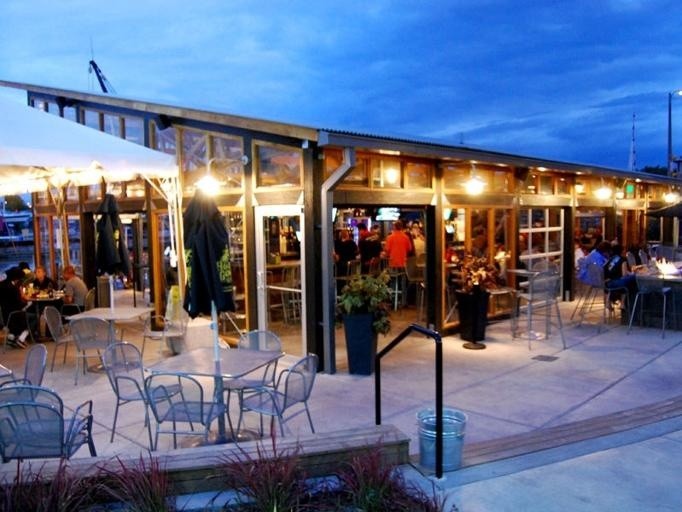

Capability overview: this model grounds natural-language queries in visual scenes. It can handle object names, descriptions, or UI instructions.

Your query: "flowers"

[456,257,498,294]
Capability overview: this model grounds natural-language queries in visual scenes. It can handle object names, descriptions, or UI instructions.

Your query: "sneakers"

[7,339,17,346]
[15,339,27,349]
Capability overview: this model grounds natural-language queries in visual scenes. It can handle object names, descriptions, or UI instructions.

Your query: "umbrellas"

[86,184,142,375]
[181,186,260,451]
[0,93,182,196]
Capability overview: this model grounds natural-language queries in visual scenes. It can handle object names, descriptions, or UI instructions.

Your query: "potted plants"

[336,268,395,376]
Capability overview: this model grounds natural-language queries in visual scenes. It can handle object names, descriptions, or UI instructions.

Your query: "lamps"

[458,159,490,197]
[193,155,250,196]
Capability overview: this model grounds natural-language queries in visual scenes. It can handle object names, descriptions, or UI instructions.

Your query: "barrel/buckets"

[415,407,468,471]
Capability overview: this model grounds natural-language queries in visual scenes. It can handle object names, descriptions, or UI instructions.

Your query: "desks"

[148,342,284,448]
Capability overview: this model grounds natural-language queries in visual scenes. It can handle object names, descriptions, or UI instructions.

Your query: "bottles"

[48,282,54,299]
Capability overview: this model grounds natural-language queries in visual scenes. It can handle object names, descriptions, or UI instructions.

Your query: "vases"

[455,290,492,349]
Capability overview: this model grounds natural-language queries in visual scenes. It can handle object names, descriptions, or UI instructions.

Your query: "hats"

[5,267,26,278]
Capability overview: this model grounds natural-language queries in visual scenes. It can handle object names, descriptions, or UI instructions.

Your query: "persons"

[31,266,53,288]
[1,265,31,347]
[57,265,90,312]
[18,262,34,279]
[573,234,631,309]
[330,217,426,307]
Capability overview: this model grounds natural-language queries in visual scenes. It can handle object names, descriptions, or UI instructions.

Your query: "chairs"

[240,354,319,437]
[0,281,194,468]
[220,252,454,336]
[215,329,282,435]
[499,244,682,356]
[144,370,235,451]
